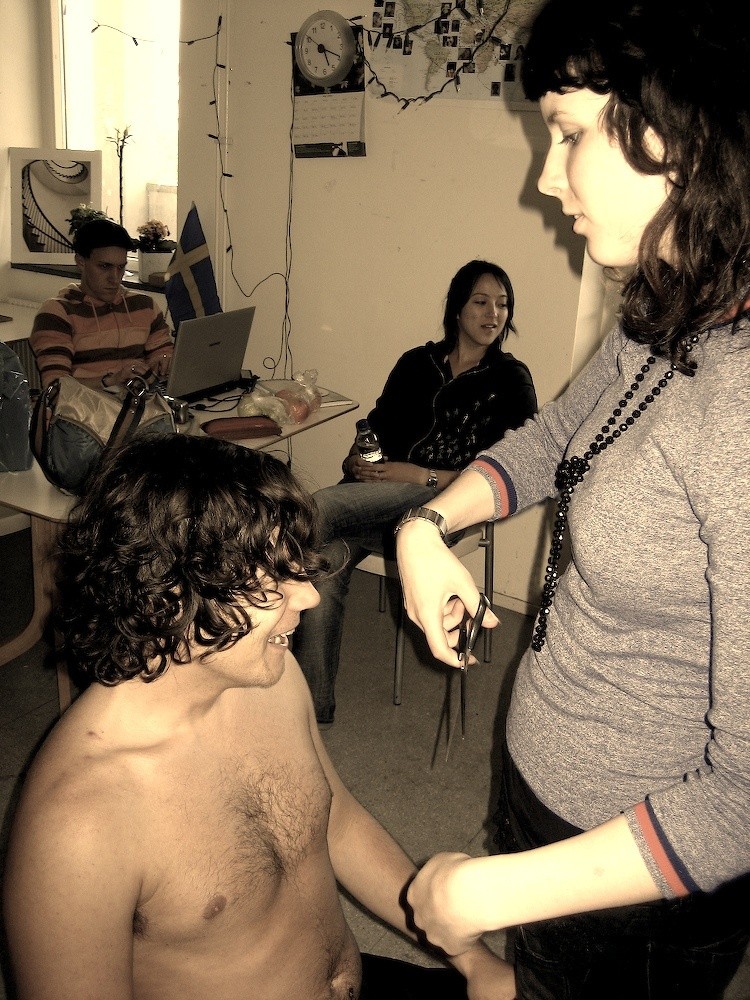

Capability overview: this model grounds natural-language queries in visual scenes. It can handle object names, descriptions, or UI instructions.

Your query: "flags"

[163,208,222,334]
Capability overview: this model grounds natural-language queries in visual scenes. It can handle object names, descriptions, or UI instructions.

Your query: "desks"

[0,378,361,713]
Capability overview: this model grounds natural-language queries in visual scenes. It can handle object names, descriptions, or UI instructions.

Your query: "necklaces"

[531,324,713,652]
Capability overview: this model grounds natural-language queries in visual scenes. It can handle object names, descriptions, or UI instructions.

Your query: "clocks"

[293,10,356,86]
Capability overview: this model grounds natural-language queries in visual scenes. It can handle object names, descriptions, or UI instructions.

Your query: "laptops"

[104,306,256,404]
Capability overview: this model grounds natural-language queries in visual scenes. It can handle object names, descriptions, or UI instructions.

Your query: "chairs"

[355,520,494,706]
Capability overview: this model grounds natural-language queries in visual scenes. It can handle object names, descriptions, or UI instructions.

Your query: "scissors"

[441,590,489,768]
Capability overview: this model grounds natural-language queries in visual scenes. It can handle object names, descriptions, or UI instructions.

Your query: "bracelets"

[102,372,115,388]
[392,506,448,540]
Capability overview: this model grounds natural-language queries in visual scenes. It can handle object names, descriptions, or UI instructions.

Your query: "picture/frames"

[8,146,102,266]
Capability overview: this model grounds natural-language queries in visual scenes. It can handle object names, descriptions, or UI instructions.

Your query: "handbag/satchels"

[0,340,33,473]
[29,372,177,498]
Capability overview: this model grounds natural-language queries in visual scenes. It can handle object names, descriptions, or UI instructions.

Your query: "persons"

[0,432,521,1000]
[371,0,523,96]
[30,218,176,392]
[290,259,538,726]
[408,0,750,1000]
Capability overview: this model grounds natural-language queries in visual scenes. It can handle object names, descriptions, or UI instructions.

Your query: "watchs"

[426,467,438,489]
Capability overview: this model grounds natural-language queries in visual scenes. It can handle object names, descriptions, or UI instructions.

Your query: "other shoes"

[317,715,334,730]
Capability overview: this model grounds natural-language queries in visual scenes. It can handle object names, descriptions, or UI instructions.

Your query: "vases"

[138,248,173,281]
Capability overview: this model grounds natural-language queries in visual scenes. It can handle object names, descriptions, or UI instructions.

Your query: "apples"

[274,388,320,422]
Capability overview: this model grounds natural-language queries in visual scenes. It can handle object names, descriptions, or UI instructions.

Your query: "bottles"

[355,419,385,464]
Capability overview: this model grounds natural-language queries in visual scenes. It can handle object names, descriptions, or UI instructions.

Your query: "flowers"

[66,202,114,234]
[129,219,178,255]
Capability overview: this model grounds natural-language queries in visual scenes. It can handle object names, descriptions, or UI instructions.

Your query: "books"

[253,377,354,407]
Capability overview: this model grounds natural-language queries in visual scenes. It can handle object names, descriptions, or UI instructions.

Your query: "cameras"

[162,395,188,424]
